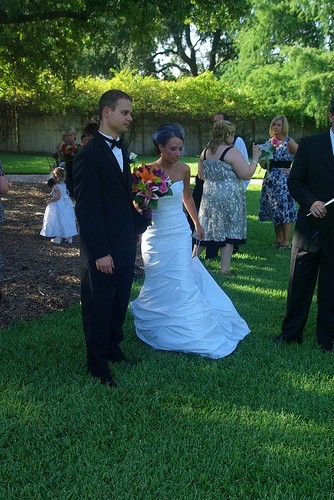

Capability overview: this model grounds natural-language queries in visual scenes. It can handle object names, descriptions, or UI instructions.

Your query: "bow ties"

[99,132,121,150]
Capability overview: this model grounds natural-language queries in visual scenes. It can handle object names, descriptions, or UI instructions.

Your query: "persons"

[72,89,147,388]
[273,96,334,354]
[186,112,251,259]
[258,114,299,249]
[38,167,78,244]
[54,123,101,188]
[192,120,263,275]
[130,121,207,359]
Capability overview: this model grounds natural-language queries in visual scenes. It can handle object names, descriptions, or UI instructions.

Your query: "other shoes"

[51,238,61,245]
[107,359,142,366]
[94,375,117,388]
[318,343,334,354]
[64,238,72,244]
[273,336,301,344]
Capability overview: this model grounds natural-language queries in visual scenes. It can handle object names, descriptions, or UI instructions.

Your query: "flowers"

[55,139,80,159]
[131,162,173,225]
[259,137,278,173]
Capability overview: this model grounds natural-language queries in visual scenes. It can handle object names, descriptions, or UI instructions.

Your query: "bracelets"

[250,158,258,166]
